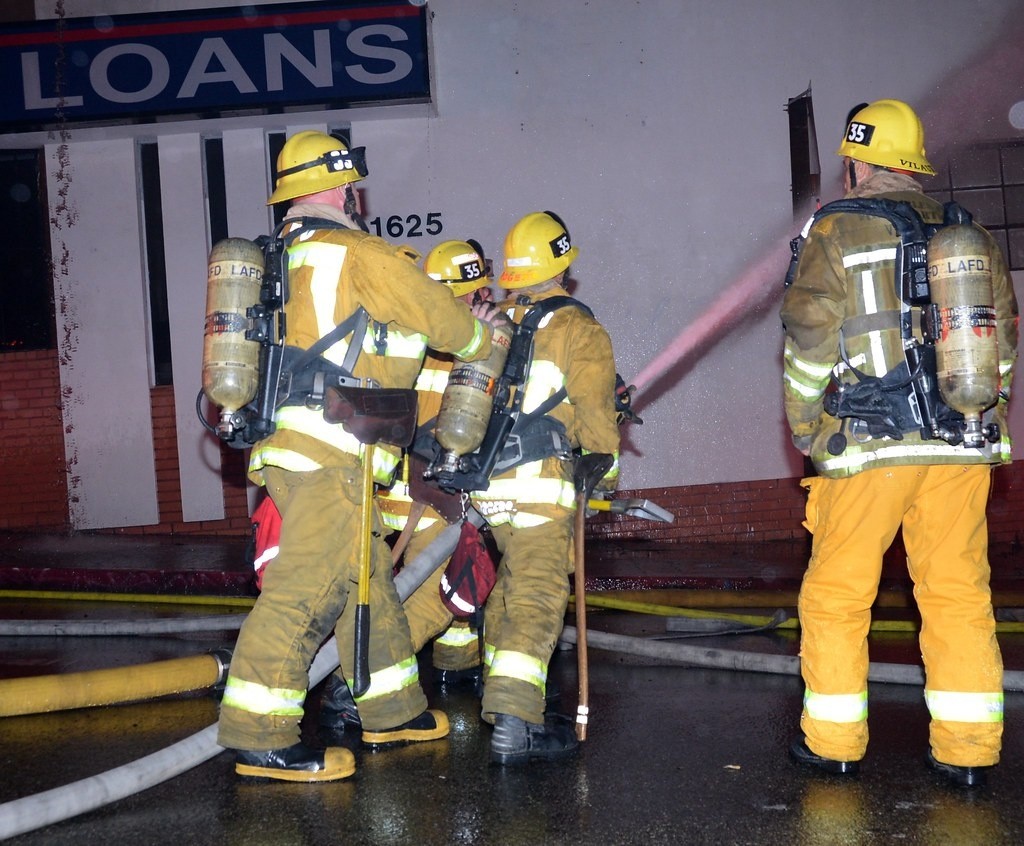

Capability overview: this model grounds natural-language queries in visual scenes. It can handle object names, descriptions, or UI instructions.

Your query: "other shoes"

[235,738,356,782]
[361,707,452,743]
[545,678,560,699]
[790,734,859,774]
[316,672,362,732]
[431,667,483,702]
[928,748,982,785]
[488,712,580,764]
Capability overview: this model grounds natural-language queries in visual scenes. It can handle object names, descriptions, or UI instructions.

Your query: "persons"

[463,213,623,763]
[778,98,1019,787]
[216,131,499,783]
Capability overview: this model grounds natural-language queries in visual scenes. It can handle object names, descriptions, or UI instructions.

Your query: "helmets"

[498,212,577,287]
[838,99,940,176]
[267,132,363,207]
[424,239,491,297]
